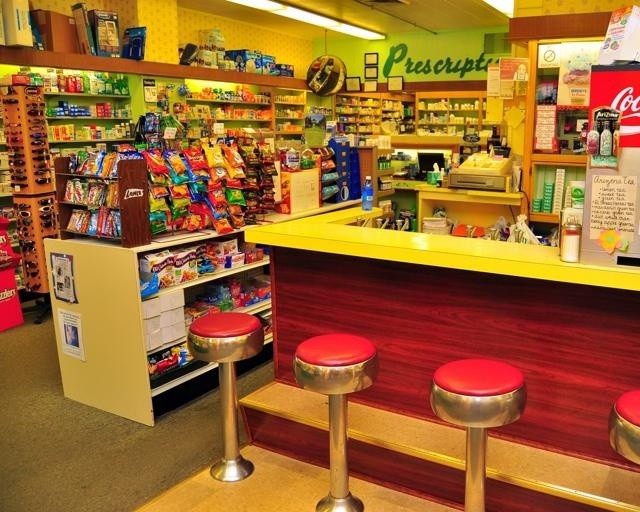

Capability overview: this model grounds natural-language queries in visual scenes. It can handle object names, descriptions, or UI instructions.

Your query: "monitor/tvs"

[418,153,445,173]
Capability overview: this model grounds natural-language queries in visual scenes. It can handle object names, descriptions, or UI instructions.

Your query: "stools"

[293,333,377,512]
[430,358,526,512]
[187,312,264,482]
[608,390,640,465]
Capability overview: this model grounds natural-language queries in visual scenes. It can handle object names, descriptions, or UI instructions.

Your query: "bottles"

[560,223,582,263]
[380,180,392,191]
[378,156,391,170]
[361,175,374,210]
[587,120,619,156]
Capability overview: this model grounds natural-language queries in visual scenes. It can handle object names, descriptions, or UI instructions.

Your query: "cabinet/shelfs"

[0,43,335,314]
[521,35,606,225]
[335,92,415,138]
[355,145,395,200]
[43,197,363,427]
[415,91,500,138]
[54,157,152,248]
